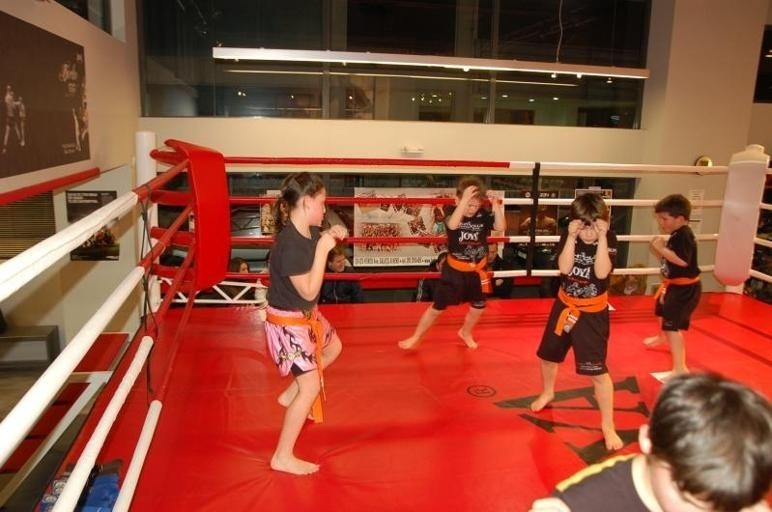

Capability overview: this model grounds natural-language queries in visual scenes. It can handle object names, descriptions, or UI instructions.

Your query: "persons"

[643,195,701,381]
[211,252,352,303]
[523,373,770,512]
[398,176,507,351]
[265,172,348,477]
[529,193,624,451]
